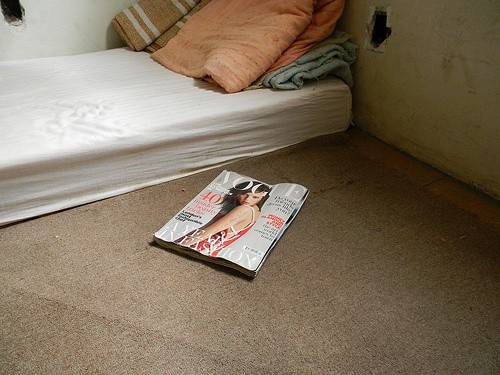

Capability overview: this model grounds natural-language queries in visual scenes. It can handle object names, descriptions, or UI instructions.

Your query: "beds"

[1,46,354,227]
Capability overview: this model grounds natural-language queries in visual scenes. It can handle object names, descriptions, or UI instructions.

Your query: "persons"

[172,181,272,258]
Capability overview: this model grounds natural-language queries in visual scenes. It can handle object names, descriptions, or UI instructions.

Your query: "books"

[152,169,310,278]
